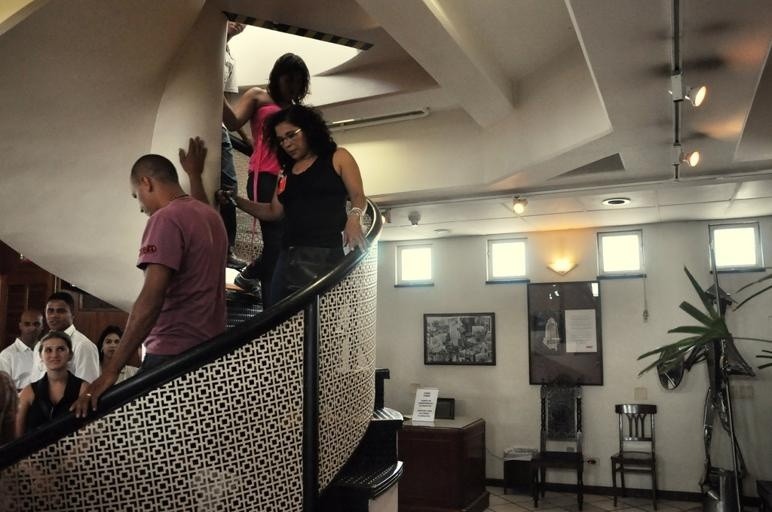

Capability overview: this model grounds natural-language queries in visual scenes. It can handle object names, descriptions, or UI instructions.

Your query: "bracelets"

[349,207,363,216]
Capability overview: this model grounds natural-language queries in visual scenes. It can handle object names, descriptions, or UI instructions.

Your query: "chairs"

[534,383,584,511]
[609,403,659,512]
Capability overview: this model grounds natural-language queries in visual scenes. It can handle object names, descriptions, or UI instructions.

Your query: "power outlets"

[584,456,600,470]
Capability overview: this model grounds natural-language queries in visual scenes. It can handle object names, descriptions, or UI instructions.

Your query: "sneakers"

[234,274,262,298]
[227,247,246,268]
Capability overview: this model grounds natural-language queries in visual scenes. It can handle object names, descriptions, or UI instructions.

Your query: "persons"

[69,136,232,420]
[223,54,311,297]
[31,292,100,384]
[15,332,91,440]
[216,105,370,304]
[0,367,16,445]
[97,324,139,386]
[223,20,250,270]
[0,307,44,395]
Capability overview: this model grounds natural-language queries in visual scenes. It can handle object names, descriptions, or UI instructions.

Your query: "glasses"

[279,128,301,145]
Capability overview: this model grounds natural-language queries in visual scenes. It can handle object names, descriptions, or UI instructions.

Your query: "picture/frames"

[525,281,603,386]
[423,312,496,366]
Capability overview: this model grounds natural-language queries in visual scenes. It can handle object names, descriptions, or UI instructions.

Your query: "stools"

[504,456,538,496]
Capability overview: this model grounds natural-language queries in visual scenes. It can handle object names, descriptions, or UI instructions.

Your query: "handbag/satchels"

[281,246,344,291]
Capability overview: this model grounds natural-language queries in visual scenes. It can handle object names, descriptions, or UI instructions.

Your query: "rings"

[84,392,92,398]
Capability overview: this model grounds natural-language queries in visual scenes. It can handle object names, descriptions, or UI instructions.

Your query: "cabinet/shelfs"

[399,417,491,511]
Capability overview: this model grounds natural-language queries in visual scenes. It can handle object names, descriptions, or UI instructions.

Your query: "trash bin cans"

[504,447,538,496]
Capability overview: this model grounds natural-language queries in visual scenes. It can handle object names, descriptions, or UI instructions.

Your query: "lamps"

[666,70,709,169]
[547,259,577,275]
[511,198,528,214]
